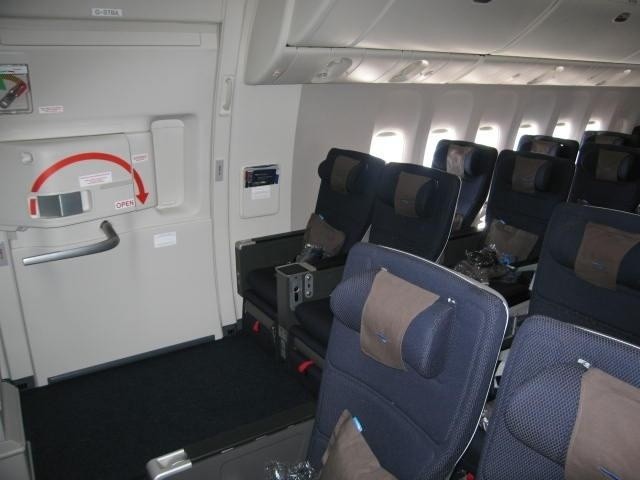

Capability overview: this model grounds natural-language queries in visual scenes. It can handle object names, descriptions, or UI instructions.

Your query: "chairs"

[503,199,636,348]
[569,134,635,213]
[146,241,512,478]
[231,145,383,367]
[446,146,569,320]
[428,136,496,248]
[469,314,638,480]
[513,134,580,161]
[271,161,463,378]
